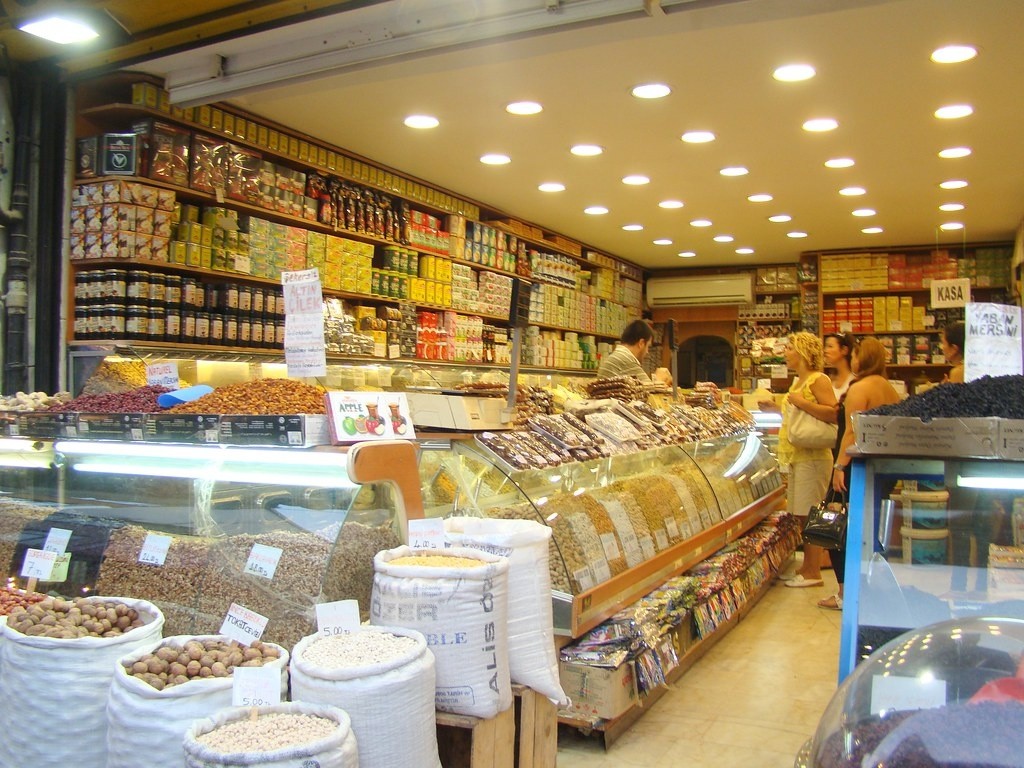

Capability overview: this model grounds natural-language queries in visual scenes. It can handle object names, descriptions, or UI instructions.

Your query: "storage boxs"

[559,660,636,721]
[0,411,331,449]
[849,411,1024,462]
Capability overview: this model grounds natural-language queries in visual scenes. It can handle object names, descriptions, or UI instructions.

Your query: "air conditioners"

[646,273,755,308]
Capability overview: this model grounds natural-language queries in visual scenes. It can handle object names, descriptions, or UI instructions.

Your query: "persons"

[597,320,654,384]
[940,323,1001,590]
[757,331,901,611]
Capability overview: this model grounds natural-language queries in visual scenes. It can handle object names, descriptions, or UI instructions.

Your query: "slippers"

[819,593,843,610]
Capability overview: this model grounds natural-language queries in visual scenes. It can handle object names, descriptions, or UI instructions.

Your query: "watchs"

[833,463,845,472]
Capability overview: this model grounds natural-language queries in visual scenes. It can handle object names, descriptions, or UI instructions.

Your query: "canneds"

[73,269,289,351]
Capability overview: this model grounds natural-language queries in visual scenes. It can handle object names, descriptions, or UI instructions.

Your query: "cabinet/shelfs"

[798,240,1016,368]
[64,69,649,375]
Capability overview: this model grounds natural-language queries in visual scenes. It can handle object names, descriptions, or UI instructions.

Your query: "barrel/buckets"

[904,480,944,491]
[901,490,949,530]
[900,528,949,570]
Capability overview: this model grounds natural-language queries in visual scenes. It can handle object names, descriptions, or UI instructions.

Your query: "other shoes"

[779,572,824,587]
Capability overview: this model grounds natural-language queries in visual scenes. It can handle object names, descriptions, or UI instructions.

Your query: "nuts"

[0,586,280,691]
[486,503,588,595]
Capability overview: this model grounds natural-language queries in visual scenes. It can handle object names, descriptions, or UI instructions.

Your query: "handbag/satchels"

[802,487,848,548]
[787,373,838,449]
